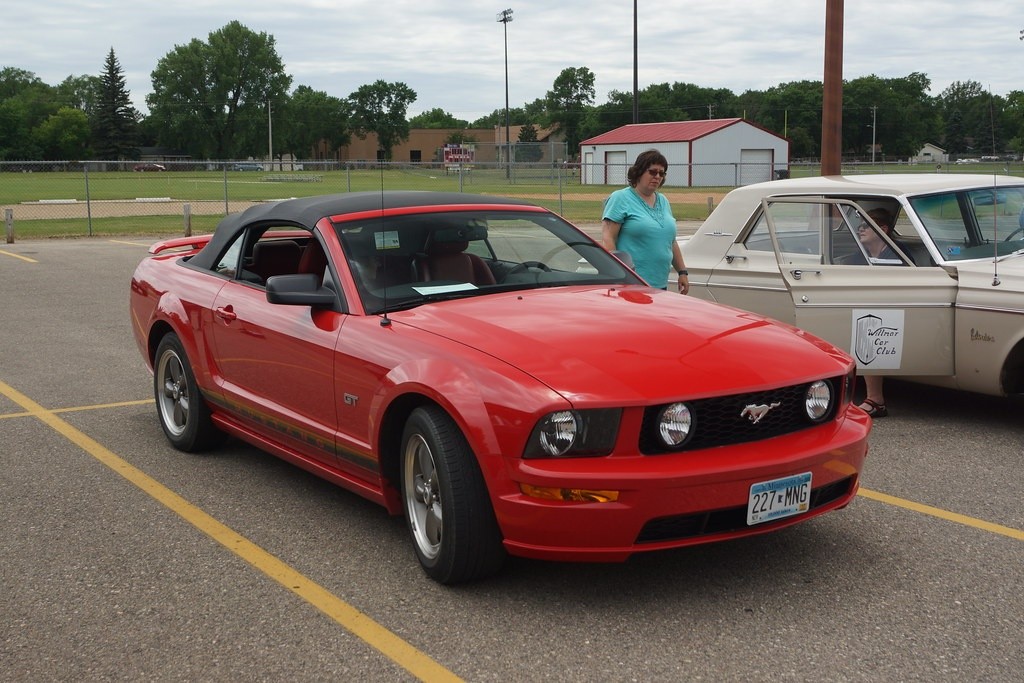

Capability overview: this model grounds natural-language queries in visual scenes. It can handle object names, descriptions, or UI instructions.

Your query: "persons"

[602,149,689,295]
[936,162,942,173]
[845,208,916,418]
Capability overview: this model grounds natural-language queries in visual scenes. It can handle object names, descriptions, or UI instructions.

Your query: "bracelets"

[678,269,688,276]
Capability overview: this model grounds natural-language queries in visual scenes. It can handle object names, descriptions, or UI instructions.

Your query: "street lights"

[867,125,875,165]
[496,9,514,178]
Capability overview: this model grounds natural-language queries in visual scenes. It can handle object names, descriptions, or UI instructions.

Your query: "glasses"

[647,169,666,177]
[858,221,869,231]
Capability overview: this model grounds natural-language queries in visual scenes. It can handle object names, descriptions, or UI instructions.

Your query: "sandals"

[857,399,887,418]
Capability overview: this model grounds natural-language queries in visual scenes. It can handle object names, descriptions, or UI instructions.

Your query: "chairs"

[412,229,498,286]
[299,232,329,276]
[908,245,934,268]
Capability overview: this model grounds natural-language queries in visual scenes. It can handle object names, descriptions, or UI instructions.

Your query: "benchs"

[253,240,410,291]
[754,233,859,256]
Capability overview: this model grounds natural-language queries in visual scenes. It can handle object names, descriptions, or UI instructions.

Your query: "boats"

[956,158,979,164]
[982,154,999,161]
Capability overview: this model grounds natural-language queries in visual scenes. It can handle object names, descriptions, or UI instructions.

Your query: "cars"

[576,173,1024,398]
[133,164,167,172]
[1000,154,1020,161]
[232,163,264,171]
[11,164,40,172]
[128,191,873,587]
[555,159,575,168]
